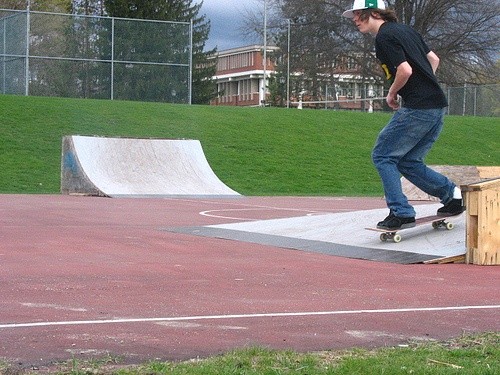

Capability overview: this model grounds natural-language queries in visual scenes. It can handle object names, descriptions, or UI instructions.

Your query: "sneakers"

[437,198,466,216]
[378,209,416,230]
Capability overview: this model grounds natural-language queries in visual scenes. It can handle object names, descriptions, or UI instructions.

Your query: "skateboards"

[364,206,465,243]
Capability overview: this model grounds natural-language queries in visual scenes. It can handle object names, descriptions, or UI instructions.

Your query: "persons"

[341,0,464,227]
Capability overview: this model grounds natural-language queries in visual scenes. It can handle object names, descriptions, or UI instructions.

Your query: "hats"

[342,0,387,18]
